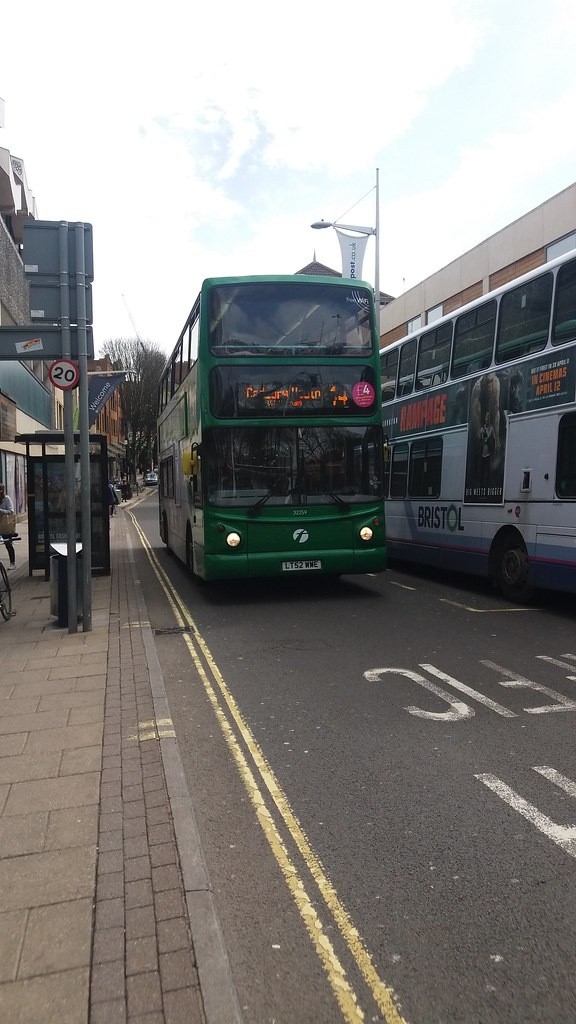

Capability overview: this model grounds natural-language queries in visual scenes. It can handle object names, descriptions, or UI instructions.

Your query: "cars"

[145,467,158,485]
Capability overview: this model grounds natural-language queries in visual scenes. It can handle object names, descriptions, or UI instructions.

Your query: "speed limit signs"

[49,360,79,390]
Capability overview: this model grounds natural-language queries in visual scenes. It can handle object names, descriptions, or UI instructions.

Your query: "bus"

[158,275,389,584]
[355,248,576,592]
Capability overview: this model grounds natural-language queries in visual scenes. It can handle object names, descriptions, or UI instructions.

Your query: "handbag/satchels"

[0,510,17,534]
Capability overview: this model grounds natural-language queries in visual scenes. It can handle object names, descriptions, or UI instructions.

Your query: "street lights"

[310,168,380,346]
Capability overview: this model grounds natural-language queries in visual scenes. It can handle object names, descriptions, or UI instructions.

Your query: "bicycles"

[0,537,21,620]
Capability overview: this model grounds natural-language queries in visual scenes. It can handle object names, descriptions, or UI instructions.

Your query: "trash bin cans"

[45,544,84,628]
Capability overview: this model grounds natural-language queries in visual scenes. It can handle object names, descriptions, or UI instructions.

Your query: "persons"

[121,481,131,502]
[0,484,16,570]
[470,373,502,474]
[108,480,119,517]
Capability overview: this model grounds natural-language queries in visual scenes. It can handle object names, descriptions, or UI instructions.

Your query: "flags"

[82,373,126,431]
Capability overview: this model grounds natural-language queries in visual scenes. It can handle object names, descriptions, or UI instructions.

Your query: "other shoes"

[8,564,16,570]
